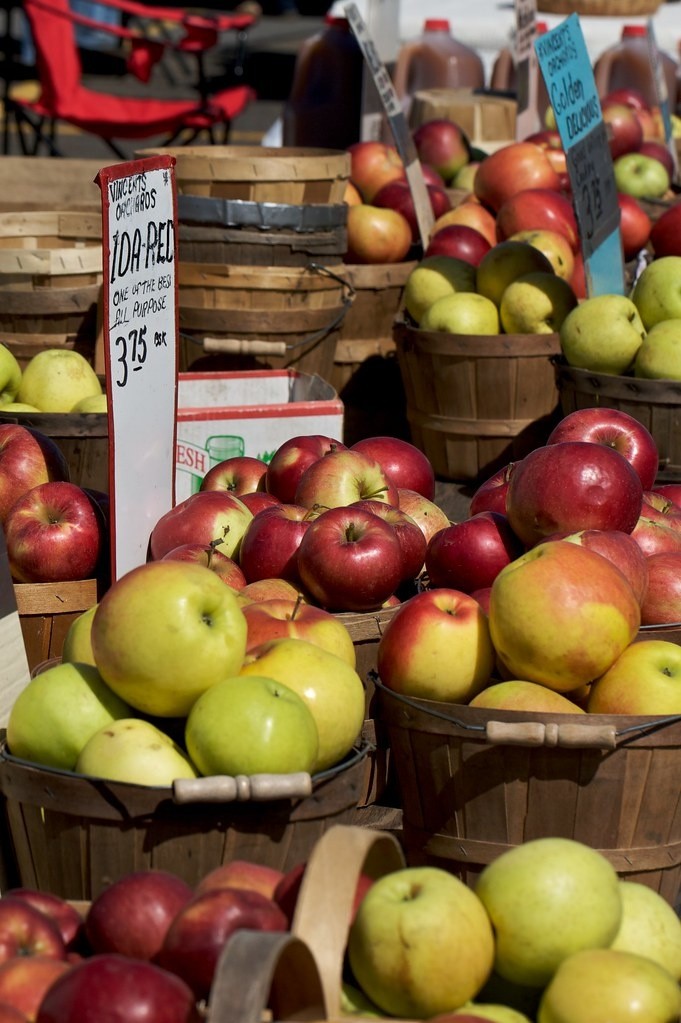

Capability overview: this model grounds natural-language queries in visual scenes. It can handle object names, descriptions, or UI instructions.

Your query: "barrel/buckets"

[0,145,681,915]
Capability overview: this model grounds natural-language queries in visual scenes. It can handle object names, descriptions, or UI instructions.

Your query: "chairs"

[0,0,255,162]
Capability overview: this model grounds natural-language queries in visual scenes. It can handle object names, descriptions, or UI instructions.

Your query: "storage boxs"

[175,368,344,503]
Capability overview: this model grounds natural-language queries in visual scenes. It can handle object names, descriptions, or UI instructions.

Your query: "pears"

[0,340,111,413]
[402,243,681,385]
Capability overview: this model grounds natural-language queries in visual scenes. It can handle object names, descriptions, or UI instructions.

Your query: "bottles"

[292,14,384,150]
[392,18,485,100]
[591,24,681,114]
[491,22,555,128]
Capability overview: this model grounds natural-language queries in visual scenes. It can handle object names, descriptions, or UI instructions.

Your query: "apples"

[0,408,681,1023]
[330,88,681,291]
